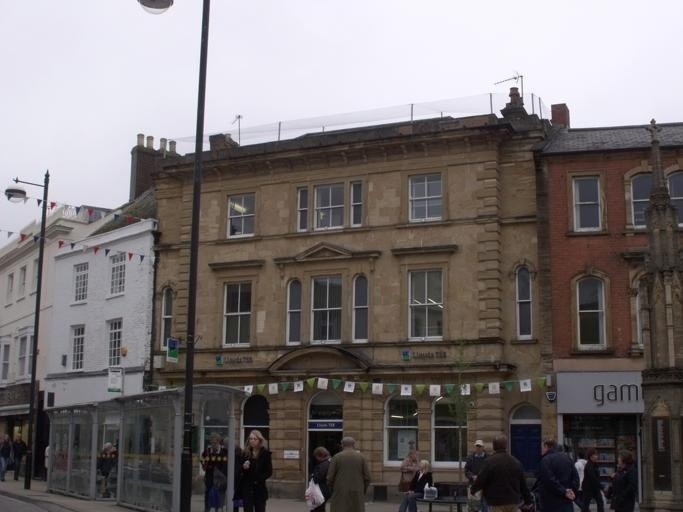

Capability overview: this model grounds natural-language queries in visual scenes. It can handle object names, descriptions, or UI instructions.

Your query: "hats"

[474,439,484,446]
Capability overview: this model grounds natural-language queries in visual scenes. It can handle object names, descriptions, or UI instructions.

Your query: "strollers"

[518,479,540,512]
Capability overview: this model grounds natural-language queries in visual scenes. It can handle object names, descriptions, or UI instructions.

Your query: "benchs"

[416,482,467,512]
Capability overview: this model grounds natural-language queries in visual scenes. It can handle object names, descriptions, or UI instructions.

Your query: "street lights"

[5,168,53,489]
[136,0,212,511]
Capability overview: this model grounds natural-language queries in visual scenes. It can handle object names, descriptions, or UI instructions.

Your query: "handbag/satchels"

[304,478,325,510]
[7,456,16,465]
[399,480,408,492]
[213,466,227,488]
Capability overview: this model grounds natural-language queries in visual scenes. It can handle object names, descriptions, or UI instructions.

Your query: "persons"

[400,449,419,495]
[0,434,14,481]
[464,435,636,512]
[399,460,432,512]
[97,442,116,498]
[309,446,332,512]
[326,437,368,512]
[13,433,27,480]
[200,430,273,512]
